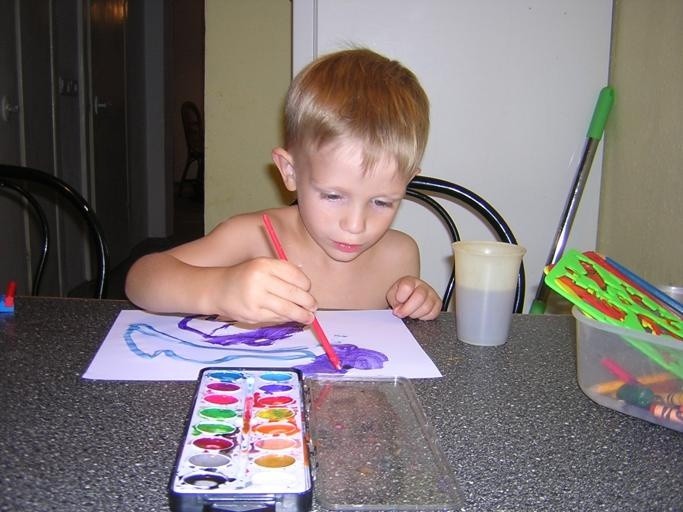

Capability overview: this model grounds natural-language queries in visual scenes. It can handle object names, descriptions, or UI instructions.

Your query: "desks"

[0,294,682,511]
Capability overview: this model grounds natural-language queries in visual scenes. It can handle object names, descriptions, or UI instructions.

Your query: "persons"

[123,42,445,327]
[307,382,407,505]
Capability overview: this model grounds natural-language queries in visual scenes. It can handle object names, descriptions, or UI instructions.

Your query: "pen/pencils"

[593,354,683,423]
[5,279,15,308]
[592,251,683,315]
[261,212,343,372]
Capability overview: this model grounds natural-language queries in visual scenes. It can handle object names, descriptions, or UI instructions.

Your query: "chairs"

[0,162,107,299]
[289,174,524,314]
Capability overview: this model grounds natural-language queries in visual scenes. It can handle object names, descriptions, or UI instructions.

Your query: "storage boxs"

[570,286,682,432]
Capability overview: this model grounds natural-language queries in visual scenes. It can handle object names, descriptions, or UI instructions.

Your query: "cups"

[451,240,526,346]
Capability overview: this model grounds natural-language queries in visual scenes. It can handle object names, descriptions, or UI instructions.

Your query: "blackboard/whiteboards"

[292,0,614,315]
